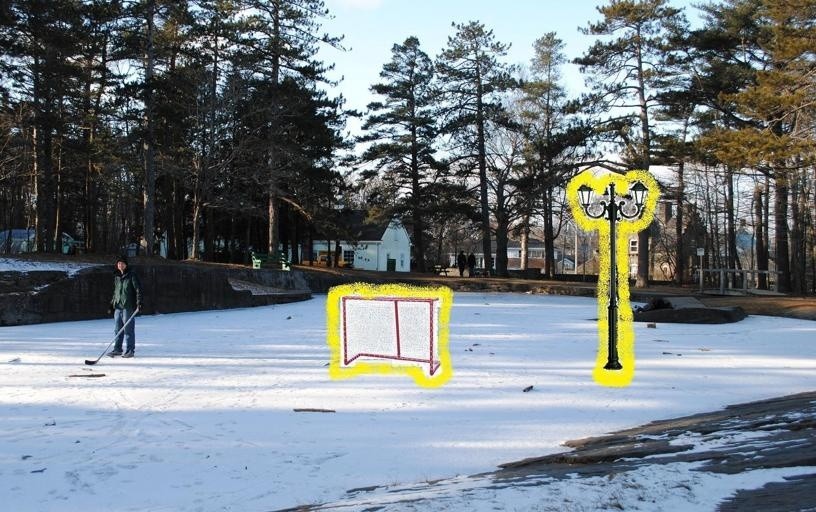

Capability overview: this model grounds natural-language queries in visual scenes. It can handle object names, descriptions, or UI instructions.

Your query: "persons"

[107,259,143,358]
[458,251,466,275]
[468,253,475,274]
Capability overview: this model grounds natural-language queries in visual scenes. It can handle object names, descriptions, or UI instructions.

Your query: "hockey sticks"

[85,309,139,364]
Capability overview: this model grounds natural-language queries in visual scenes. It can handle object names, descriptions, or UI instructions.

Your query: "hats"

[115,257,128,266]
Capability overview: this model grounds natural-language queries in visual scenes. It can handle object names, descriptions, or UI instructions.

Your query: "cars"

[10,228,87,255]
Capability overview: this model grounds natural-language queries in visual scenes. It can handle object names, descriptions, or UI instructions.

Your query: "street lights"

[578,180,650,372]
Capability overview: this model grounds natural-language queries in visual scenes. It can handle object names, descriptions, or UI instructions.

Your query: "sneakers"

[121,349,134,358]
[108,349,123,355]
